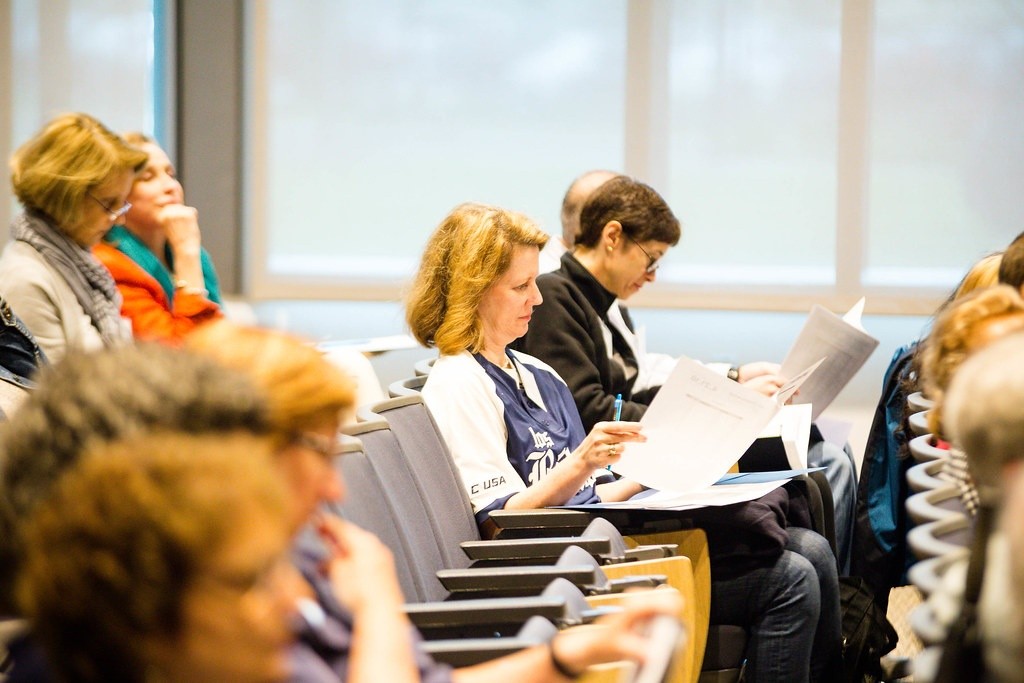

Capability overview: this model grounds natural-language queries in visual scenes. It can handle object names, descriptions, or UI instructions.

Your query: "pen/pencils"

[608,394,622,470]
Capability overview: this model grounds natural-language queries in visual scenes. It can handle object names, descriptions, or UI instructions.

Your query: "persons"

[0,113,1024,683]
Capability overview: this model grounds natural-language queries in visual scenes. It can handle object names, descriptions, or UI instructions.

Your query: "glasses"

[629,234,659,274]
[84,190,133,222]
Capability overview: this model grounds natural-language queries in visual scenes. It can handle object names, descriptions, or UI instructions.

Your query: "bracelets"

[549,632,585,680]
[172,280,208,296]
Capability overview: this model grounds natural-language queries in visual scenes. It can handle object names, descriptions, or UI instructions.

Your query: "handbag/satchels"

[838,575,899,683]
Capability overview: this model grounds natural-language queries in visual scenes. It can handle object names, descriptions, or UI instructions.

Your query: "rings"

[609,445,615,456]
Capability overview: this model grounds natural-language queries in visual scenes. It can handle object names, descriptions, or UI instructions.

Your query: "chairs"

[322,335,970,683]
